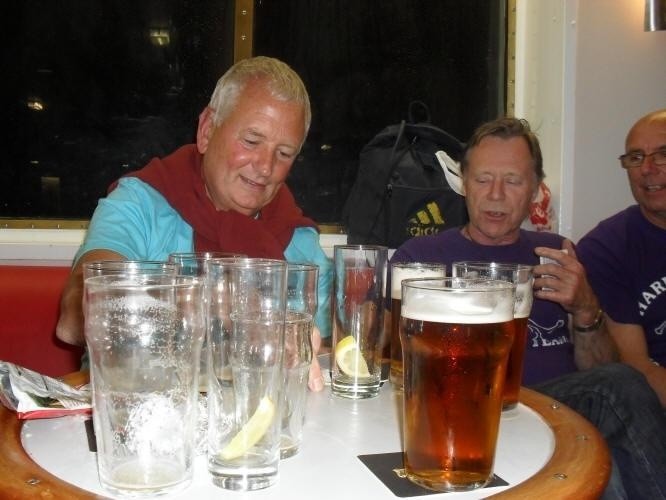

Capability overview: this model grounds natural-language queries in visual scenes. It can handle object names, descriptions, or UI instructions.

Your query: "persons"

[57,56,334,393]
[576,109,666,409]
[381,117,665,500]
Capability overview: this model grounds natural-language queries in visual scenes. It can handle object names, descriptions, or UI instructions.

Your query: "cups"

[82,252,319,497]
[392,259,533,489]
[331,243,390,402]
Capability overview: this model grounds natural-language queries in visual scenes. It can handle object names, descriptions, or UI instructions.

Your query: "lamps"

[643,0,666,33]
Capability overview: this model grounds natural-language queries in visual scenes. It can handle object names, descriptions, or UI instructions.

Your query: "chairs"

[0,263,84,377]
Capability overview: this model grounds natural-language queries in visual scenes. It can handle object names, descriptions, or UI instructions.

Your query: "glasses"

[618,148,666,171]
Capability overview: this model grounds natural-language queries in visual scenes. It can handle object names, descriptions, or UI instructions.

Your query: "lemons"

[334,335,372,378]
[220,394,278,461]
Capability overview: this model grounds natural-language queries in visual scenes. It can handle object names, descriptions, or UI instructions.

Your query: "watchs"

[573,309,603,332]
